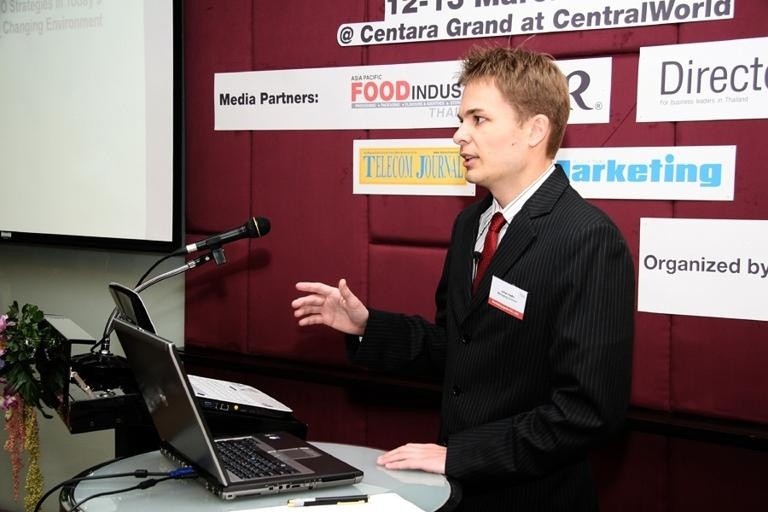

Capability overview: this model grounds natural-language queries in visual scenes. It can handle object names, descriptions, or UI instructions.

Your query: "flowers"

[1,295,65,511]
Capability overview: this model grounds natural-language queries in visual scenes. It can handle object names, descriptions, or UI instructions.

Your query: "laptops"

[108,282,293,420]
[112,318,363,501]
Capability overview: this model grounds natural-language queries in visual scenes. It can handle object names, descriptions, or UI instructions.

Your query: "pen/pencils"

[287,495,368,507]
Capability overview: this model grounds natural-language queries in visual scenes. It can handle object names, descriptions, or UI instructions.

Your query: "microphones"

[185,216,271,254]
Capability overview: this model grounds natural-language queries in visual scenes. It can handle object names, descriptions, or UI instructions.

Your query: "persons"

[290,41,637,510]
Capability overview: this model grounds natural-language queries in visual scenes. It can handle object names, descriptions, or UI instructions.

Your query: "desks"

[57,432,461,509]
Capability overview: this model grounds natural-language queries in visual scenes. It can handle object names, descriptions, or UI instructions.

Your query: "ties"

[473,213,507,293]
